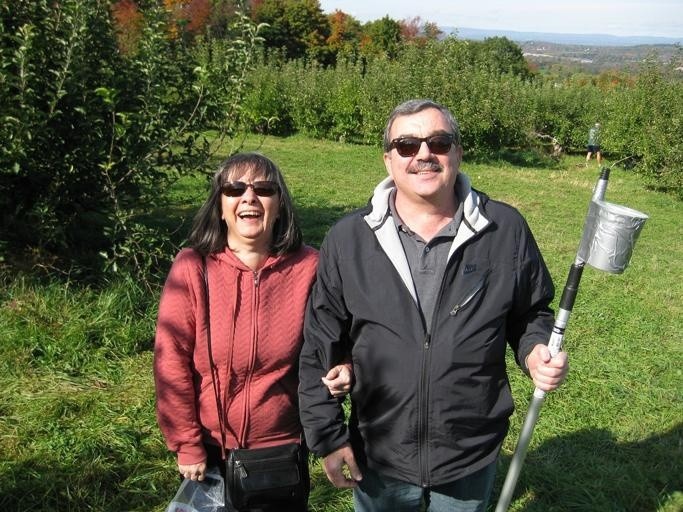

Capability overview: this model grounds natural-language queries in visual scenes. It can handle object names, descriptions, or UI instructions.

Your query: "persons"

[298,100,568,512]
[582,115,606,170]
[152,153,352,512]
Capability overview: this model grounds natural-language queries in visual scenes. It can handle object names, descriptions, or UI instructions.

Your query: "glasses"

[387,133,457,157]
[218,180,278,198]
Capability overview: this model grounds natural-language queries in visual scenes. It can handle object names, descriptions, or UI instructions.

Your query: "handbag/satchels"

[223,441,311,512]
